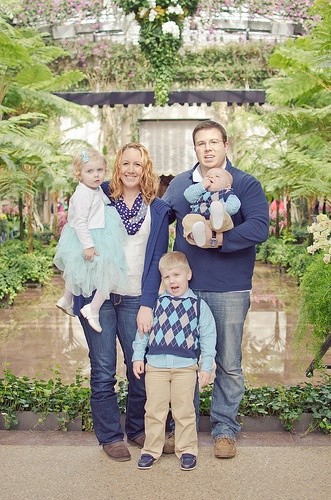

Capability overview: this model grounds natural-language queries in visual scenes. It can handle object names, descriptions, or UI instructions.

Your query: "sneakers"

[214,438,236,458]
[127,433,146,448]
[179,453,197,470]
[162,433,175,454]
[102,440,131,461]
[137,454,158,469]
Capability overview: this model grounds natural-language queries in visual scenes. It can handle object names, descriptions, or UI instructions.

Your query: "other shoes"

[80,303,102,333]
[211,201,225,230]
[56,296,76,317]
[192,221,206,248]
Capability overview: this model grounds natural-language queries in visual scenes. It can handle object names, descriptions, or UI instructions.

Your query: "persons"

[181,168,241,249]
[159,119,270,458]
[131,251,218,472]
[56,150,126,332]
[72,142,176,462]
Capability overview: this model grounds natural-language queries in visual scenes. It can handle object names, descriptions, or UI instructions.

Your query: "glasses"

[196,139,224,150]
